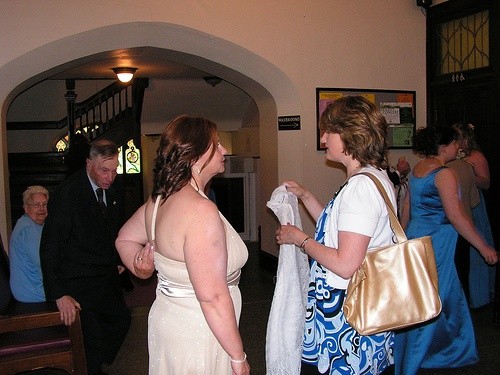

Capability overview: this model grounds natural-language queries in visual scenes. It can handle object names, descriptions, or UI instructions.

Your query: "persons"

[114,114,253,375]
[264,95,500,375]
[8,136,127,375]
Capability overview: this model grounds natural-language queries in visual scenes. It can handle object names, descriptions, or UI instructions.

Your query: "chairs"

[0,232,88,375]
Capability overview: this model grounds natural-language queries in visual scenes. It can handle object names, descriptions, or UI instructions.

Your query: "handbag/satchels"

[333,172,443,336]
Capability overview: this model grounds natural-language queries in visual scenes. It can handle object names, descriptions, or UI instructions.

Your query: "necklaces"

[427,154,448,167]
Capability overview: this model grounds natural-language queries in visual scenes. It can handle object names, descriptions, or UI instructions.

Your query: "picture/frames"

[316,87,417,151]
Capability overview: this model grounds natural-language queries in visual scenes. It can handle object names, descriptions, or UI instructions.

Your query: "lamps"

[112,68,137,83]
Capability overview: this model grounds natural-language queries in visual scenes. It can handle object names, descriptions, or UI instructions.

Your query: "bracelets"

[298,235,312,251]
[227,351,247,365]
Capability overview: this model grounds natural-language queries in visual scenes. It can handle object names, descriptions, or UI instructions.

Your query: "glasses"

[25,201,47,209]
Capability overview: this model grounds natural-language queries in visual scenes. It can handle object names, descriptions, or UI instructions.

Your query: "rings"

[136,256,143,262]
